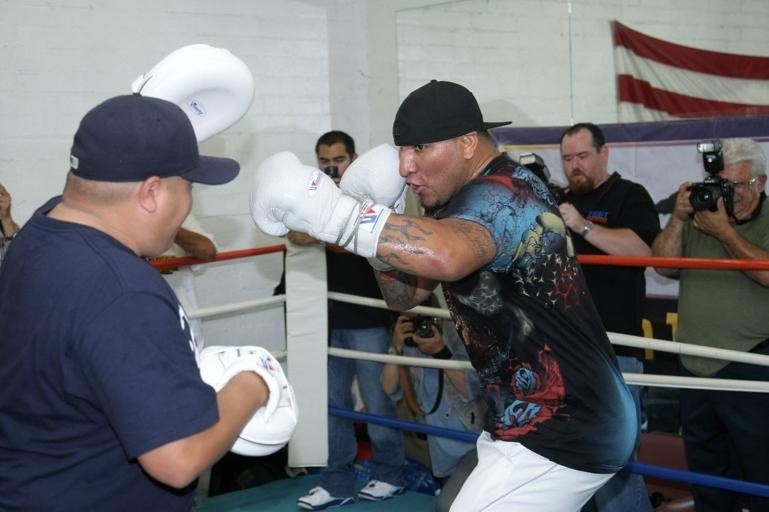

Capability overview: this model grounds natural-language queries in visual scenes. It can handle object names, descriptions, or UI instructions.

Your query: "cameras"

[323,164,339,183]
[400,314,442,350]
[518,152,565,206]
[684,137,735,229]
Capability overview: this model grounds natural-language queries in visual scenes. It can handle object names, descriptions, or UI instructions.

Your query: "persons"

[0,45,299,511]
[0,185,20,256]
[147,213,219,504]
[284,131,406,510]
[650,139,768,512]
[379,300,490,487]
[253,81,640,512]
[552,123,662,512]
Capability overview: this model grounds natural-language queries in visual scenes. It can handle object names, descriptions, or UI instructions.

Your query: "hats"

[69,94,240,185]
[391,78,512,146]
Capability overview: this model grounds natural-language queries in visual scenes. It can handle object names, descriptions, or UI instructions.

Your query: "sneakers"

[297,485,356,511]
[358,480,405,502]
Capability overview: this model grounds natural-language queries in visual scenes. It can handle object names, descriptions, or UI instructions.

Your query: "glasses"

[732,176,758,190]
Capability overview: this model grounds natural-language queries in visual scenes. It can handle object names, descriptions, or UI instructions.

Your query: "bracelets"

[432,347,453,362]
[5,225,19,242]
[581,219,594,238]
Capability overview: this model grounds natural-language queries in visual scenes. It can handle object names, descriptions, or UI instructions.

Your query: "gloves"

[199,343,298,457]
[252,144,410,273]
[132,44,255,143]
